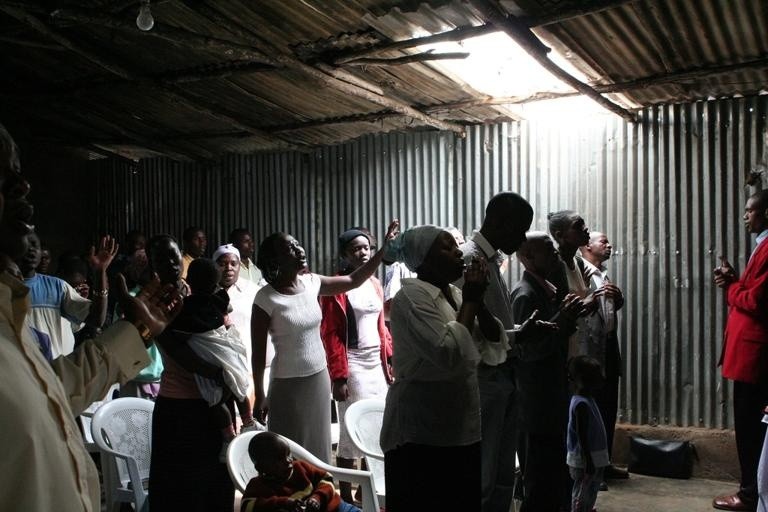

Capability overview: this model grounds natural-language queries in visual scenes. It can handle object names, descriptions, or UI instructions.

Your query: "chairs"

[80,383,120,511]
[226,431,381,511]
[344,397,388,496]
[90,396,155,512]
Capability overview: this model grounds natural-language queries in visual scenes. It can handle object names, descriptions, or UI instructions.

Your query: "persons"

[0,124,184,512]
[576,232,629,491]
[461,193,560,512]
[187,226,205,255]
[383,259,417,324]
[162,257,257,464]
[142,236,235,512]
[19,232,119,361]
[39,244,51,273]
[439,227,466,250]
[251,219,400,464]
[241,432,365,512]
[712,189,767,512]
[510,232,583,512]
[549,210,601,369]
[566,355,610,512]
[353,227,378,259]
[230,228,270,289]
[319,230,396,504]
[212,242,274,412]
[379,227,510,512]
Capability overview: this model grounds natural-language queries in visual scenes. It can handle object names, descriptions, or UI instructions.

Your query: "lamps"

[137,1,154,31]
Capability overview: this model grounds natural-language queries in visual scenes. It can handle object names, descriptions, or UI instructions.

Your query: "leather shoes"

[604,463,632,479]
[713,493,757,511]
[599,481,608,491]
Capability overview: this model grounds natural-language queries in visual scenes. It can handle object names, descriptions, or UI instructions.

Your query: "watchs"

[124,315,151,342]
[91,288,108,296]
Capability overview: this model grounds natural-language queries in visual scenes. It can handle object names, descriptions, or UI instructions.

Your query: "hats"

[384,225,442,272]
[336,228,371,259]
[211,242,241,264]
